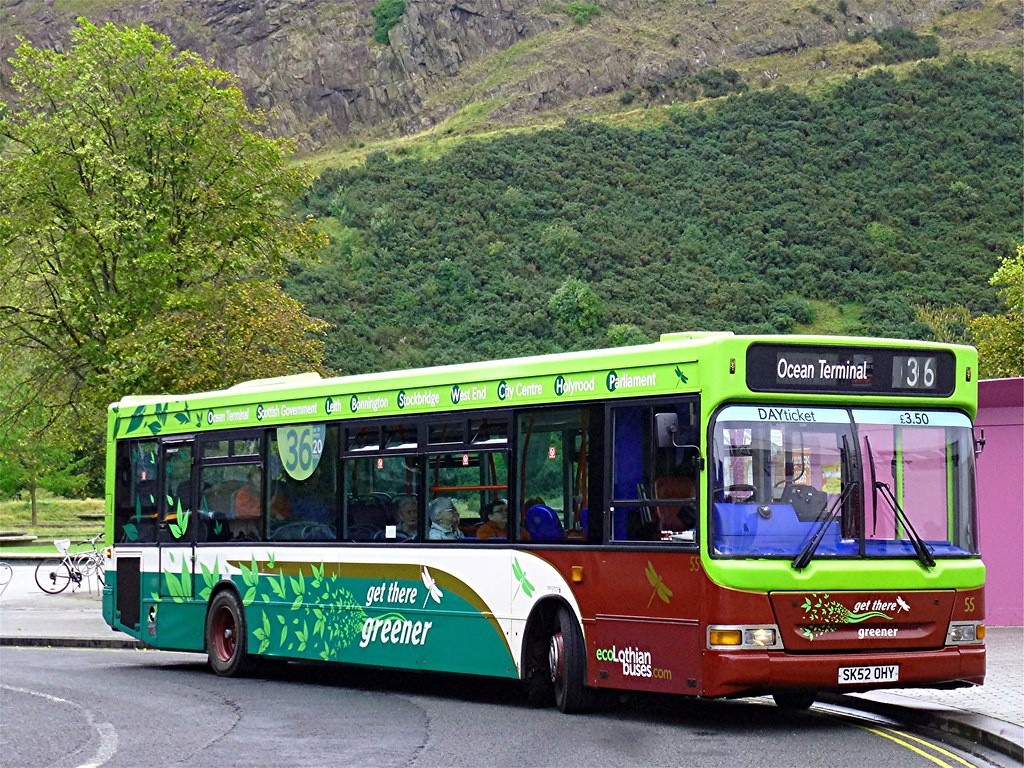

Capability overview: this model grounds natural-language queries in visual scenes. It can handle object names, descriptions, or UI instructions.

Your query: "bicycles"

[35,532,105,595]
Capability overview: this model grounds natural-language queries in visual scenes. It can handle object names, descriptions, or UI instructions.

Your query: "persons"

[395,497,418,540]
[428,497,466,540]
[520,496,545,528]
[236,465,293,522]
[476,500,531,540]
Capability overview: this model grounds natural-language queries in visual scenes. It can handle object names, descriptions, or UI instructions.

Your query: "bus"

[102,331,986,718]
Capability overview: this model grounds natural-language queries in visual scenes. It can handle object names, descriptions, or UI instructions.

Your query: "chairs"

[135,478,417,541]
[525,504,569,542]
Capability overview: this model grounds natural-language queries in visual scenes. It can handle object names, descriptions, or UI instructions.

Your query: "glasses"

[492,509,507,513]
[444,508,457,511]
